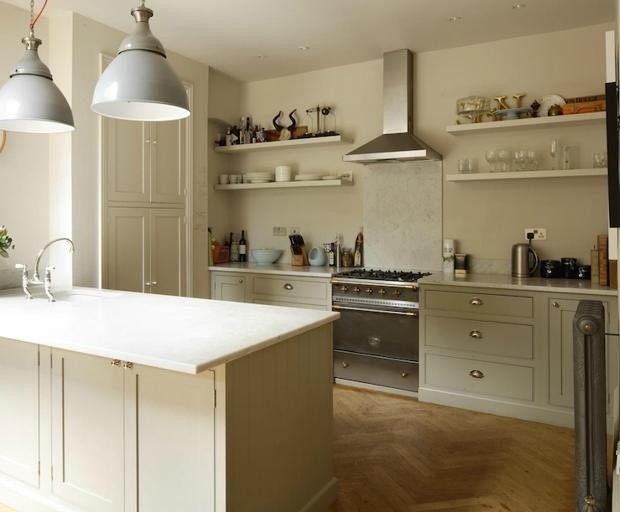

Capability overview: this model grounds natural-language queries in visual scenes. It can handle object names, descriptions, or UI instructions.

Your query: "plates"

[243,173,273,183]
[323,175,341,180]
[294,172,327,181]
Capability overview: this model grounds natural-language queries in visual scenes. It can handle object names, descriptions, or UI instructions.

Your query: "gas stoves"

[332,269,431,307]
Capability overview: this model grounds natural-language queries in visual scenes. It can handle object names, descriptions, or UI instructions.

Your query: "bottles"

[354,245,362,266]
[561,258,578,278]
[230,239,239,262]
[341,248,352,267]
[239,230,247,262]
[226,115,267,145]
[329,243,335,266]
[214,240,229,264]
[542,260,561,278]
[577,266,591,279]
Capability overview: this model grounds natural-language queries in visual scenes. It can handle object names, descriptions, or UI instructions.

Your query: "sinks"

[37,290,104,304]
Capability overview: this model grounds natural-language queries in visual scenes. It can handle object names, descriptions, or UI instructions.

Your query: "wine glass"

[515,151,526,172]
[486,151,498,172]
[528,151,539,171]
[499,151,510,172]
[549,140,561,171]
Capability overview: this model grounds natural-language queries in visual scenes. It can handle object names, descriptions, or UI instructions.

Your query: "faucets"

[16,237,75,302]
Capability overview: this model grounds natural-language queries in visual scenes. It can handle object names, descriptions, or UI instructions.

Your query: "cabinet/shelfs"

[418,286,619,434]
[445,111,608,181]
[207,274,331,311]
[104,208,186,299]
[105,79,194,205]
[0,339,50,512]
[214,136,353,189]
[51,348,214,512]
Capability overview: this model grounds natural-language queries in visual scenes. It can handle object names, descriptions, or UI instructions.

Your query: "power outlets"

[525,228,547,241]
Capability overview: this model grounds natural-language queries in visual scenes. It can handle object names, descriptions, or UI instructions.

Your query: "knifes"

[288,233,307,266]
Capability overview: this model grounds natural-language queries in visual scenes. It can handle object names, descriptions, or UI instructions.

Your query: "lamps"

[90,0,191,122]
[0,1,76,133]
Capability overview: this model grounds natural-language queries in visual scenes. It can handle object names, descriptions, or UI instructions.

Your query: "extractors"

[341,49,443,167]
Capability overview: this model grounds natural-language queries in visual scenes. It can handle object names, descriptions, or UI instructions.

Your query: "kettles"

[512,243,539,278]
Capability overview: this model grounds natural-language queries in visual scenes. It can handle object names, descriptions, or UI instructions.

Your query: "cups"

[236,174,241,183]
[229,174,235,184]
[275,166,291,181]
[219,174,228,184]
[458,159,467,173]
[562,146,571,170]
[468,158,475,173]
[594,152,607,168]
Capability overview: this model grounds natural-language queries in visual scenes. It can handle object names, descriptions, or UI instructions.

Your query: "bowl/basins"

[251,249,282,264]
